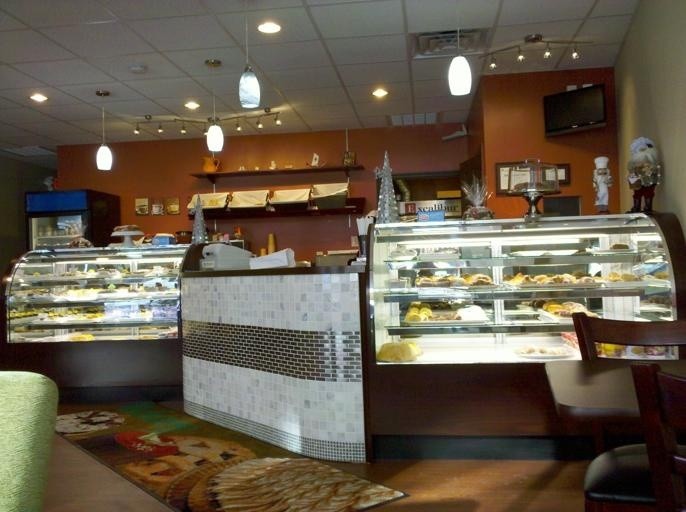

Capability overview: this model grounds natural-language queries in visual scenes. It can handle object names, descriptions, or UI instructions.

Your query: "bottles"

[35,225,78,250]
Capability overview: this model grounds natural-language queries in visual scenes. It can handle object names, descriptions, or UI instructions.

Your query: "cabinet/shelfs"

[186,165,366,219]
[5,240,211,404]
[364,211,686,460]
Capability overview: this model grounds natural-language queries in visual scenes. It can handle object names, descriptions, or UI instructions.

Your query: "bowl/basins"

[175,231,191,244]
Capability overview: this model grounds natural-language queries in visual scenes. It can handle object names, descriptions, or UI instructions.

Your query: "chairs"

[628,361,686,512]
[571,313,686,511]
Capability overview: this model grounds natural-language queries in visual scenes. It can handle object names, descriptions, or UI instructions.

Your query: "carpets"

[54,402,410,511]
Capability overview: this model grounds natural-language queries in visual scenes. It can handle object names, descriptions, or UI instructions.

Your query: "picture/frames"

[541,163,571,186]
[495,161,537,195]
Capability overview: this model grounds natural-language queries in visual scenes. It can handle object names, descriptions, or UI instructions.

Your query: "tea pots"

[200,157,220,173]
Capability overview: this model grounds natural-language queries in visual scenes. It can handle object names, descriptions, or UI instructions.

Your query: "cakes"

[5,263,182,340]
[372,241,671,363]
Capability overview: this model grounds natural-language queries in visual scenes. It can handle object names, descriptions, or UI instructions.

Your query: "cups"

[267,232,275,255]
[233,224,241,239]
[405,203,416,213]
[224,233,230,241]
[259,248,266,256]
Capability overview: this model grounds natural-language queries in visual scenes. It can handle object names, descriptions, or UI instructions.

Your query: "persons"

[591,154,616,215]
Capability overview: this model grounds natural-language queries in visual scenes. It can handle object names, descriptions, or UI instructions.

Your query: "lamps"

[238,0,260,109]
[132,111,282,136]
[447,0,472,96]
[205,59,224,153]
[95,91,113,170]
[478,34,593,71]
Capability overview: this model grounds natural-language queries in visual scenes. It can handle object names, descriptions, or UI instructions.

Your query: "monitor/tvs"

[543,83,607,136]
[543,196,581,216]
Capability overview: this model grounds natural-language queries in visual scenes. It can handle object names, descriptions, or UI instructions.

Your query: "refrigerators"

[24,190,121,251]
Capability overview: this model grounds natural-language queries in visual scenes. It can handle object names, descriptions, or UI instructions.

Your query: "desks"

[544,358,685,421]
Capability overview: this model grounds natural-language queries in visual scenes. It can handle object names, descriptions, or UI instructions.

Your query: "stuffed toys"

[625,136,664,214]
